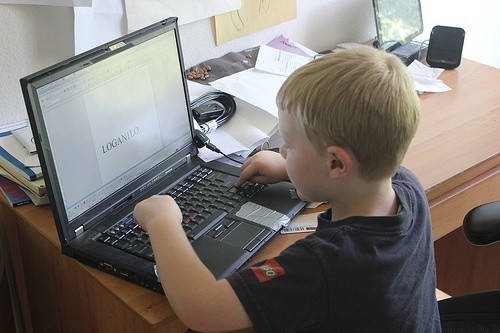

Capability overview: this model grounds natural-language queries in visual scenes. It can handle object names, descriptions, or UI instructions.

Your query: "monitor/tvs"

[372,0,423,53]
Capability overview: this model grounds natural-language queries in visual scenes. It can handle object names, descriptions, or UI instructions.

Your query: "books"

[0,36,340,208]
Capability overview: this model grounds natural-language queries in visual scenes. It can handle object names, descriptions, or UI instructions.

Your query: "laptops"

[19,17,310,298]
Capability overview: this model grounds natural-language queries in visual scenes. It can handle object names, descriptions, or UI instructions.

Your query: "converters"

[192,103,224,122]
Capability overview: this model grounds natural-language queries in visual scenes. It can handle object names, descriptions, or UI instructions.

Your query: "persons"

[133,44,441,333]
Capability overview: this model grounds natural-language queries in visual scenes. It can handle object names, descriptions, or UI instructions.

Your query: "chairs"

[438,199,500,333]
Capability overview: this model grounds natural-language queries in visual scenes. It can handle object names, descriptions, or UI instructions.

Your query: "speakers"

[426,25,465,68]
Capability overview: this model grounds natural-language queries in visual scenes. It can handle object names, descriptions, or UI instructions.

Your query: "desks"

[0,39,500,333]
